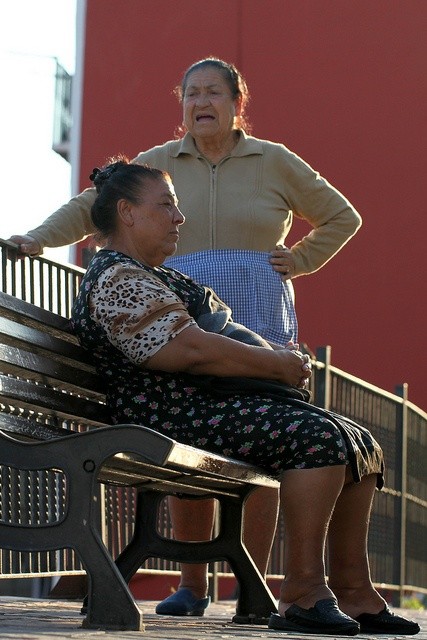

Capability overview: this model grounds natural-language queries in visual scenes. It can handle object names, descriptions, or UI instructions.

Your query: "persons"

[1,58,364,617]
[71,154,420,636]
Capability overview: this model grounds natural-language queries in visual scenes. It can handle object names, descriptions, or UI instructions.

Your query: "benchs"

[0,291,280,631]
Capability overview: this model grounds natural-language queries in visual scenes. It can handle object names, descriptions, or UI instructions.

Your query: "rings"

[286,266,289,273]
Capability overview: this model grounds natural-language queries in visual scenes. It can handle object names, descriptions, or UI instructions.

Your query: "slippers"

[268,598,360,635]
[156,587,208,615]
[353,604,420,635]
